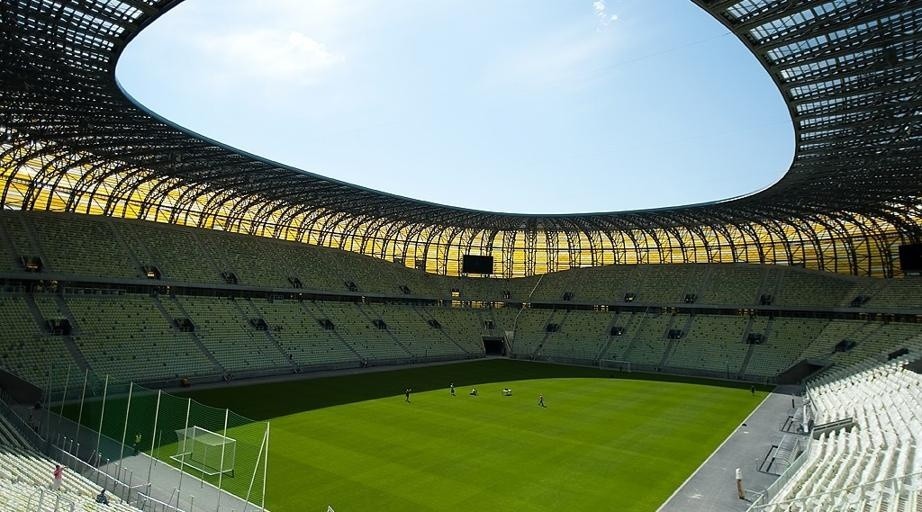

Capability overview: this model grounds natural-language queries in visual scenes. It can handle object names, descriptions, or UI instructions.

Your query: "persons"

[751,385,755,396]
[360,358,367,368]
[537,393,544,407]
[96,490,109,506]
[470,388,477,396]
[133,431,142,456]
[34,399,42,409]
[405,389,412,402]
[54,464,68,491]
[450,383,456,396]
